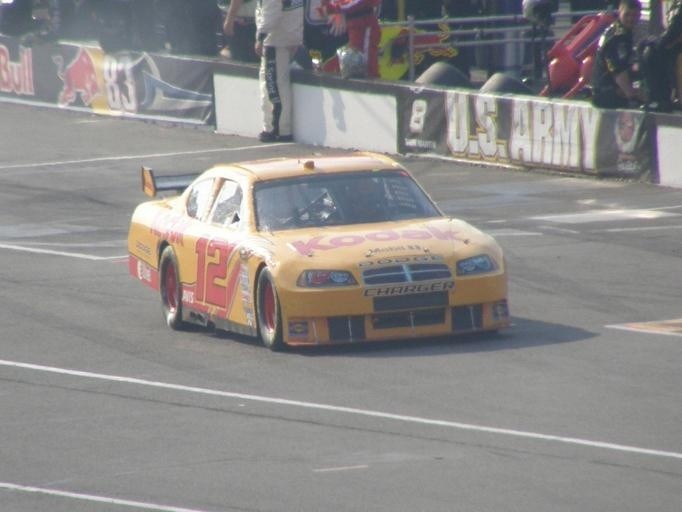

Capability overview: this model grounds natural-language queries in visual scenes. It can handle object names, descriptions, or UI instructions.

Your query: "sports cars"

[126,151,512,352]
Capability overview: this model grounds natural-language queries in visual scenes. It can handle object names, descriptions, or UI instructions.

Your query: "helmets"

[351,178,382,203]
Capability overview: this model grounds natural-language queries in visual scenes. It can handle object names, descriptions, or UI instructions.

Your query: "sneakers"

[259,131,292,141]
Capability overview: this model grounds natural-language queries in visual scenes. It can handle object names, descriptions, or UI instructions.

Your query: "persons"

[637,6,682,112]
[590,0,642,109]
[327,0,381,77]
[223,0,304,142]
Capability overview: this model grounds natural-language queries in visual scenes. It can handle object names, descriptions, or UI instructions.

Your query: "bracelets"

[226,16,234,20]
